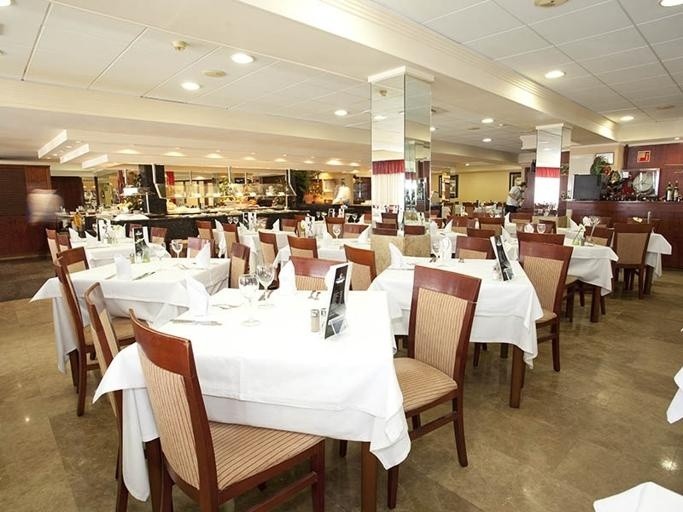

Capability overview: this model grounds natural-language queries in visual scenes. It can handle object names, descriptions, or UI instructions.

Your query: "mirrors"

[404,74,432,212]
[560,128,572,209]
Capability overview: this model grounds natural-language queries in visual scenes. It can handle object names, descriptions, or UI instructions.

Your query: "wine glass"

[316,212,327,221]
[172,239,184,265]
[346,214,357,223]
[332,223,342,241]
[227,216,239,224]
[153,241,166,271]
[238,272,261,328]
[255,264,275,311]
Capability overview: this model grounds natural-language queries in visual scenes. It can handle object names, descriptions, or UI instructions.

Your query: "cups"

[524,224,546,233]
[589,215,600,225]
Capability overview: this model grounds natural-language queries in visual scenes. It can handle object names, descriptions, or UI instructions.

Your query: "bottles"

[310,307,325,333]
[136,251,142,264]
[673,180,680,201]
[665,181,672,202]
[130,252,135,264]
[492,267,500,280]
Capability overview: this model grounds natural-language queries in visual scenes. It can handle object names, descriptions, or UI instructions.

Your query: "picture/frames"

[509,172,520,189]
[595,152,614,165]
[439,176,458,198]
[637,150,651,164]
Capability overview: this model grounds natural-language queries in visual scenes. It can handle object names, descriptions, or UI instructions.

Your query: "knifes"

[170,318,221,327]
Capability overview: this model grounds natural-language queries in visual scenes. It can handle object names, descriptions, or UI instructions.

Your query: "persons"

[331,181,351,207]
[504,181,528,214]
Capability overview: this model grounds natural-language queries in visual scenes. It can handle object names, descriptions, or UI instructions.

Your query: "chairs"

[46,201,661,512]
